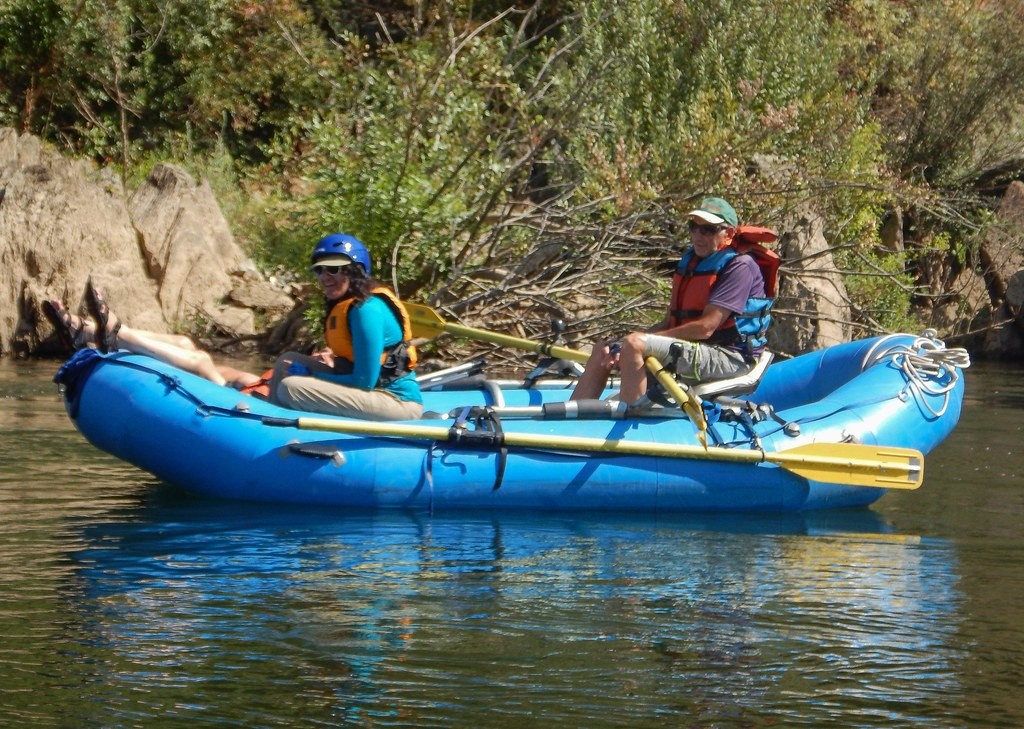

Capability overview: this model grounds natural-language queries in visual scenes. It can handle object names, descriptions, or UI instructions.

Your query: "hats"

[688,197,737,230]
[310,255,353,272]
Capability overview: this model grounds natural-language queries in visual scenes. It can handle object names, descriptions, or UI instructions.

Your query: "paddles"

[259,413,925,493]
[402,300,593,362]
[645,357,709,449]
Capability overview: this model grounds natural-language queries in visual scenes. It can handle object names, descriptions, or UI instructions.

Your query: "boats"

[51,332,965,514]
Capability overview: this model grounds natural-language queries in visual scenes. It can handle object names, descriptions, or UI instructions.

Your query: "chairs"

[691,347,774,400]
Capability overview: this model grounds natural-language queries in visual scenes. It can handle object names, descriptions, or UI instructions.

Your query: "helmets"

[311,234,371,277]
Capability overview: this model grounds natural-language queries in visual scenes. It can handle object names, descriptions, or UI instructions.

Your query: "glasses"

[686,220,728,236]
[313,265,350,274]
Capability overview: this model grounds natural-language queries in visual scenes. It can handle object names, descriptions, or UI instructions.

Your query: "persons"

[268,232,424,423]
[568,197,769,406]
